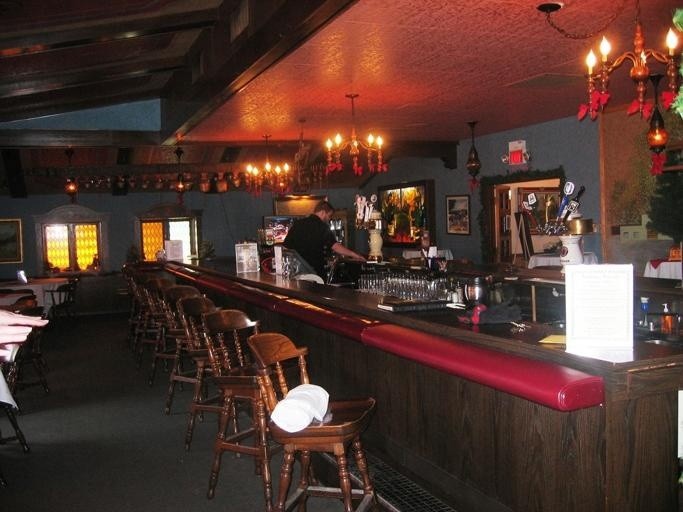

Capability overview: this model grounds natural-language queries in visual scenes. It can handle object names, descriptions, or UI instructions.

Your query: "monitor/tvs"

[262,214,306,246]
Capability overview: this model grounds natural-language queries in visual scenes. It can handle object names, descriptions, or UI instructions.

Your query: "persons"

[0,307,50,358]
[283,200,367,282]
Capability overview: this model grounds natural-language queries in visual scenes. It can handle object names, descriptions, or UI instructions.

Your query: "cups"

[282,256,300,281]
[355,273,463,305]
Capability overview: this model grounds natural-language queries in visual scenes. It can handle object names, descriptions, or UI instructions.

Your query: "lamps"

[536,0,679,120]
[63,147,77,195]
[648,72,668,157]
[173,147,185,192]
[244,93,389,198]
[465,121,482,191]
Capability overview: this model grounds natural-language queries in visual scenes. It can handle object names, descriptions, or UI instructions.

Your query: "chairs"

[0,276,82,487]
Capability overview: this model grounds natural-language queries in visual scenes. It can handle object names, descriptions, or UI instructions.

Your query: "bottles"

[329,219,344,243]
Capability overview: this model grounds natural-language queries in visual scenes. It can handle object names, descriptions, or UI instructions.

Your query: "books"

[378,298,450,312]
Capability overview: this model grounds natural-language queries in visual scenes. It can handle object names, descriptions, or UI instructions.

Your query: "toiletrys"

[660,303,672,333]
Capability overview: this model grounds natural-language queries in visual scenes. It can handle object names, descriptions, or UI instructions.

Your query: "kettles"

[463,273,489,310]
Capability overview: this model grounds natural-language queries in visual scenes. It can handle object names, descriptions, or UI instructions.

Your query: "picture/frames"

[377,178,436,248]
[444,194,471,235]
[0,218,24,265]
